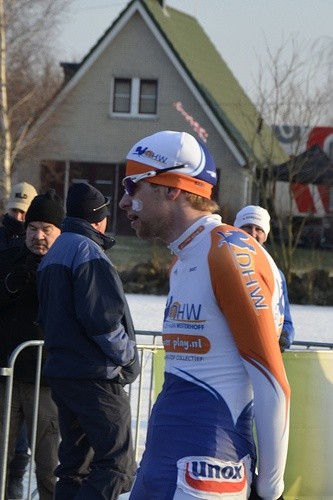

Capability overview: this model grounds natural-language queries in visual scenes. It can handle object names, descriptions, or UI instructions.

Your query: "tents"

[255,142,332,207]
[254,142,333,250]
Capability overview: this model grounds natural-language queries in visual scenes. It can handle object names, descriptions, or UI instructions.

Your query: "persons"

[0,189,68,500]
[37,180,143,500]
[0,181,38,500]
[233,206,294,354]
[118,131,291,500]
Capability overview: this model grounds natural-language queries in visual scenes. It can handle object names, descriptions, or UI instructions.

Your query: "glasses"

[122,163,189,198]
[92,195,112,213]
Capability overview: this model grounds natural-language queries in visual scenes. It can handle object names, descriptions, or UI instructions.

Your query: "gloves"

[279,331,289,352]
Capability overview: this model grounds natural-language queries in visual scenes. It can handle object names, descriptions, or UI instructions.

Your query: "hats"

[24,188,64,231]
[233,205,270,241]
[126,130,217,200]
[6,182,38,212]
[66,182,112,226]
